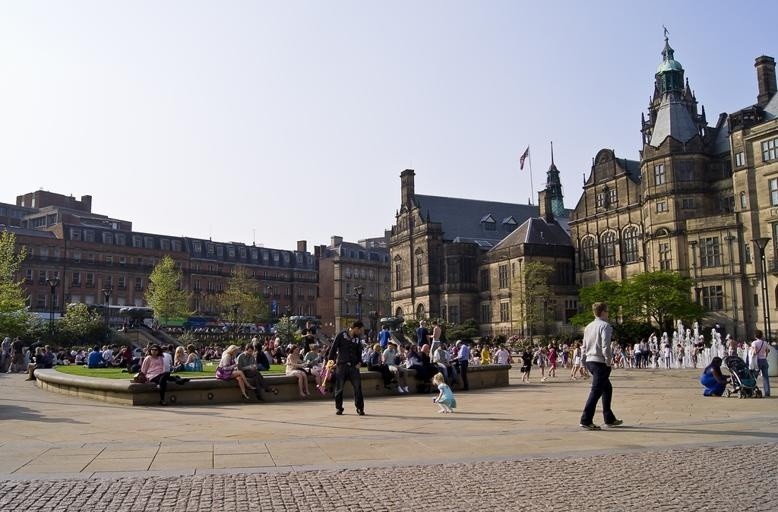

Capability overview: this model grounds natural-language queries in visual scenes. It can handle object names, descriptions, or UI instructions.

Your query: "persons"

[580,302,622,431]
[0,337,54,382]
[449,339,585,391]
[217,317,457,415]
[52,342,222,384]
[701,357,731,396]
[751,330,770,396]
[742,341,749,349]
[726,334,739,357]
[612,343,698,369]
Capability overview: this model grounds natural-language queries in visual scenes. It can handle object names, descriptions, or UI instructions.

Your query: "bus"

[83,303,154,326]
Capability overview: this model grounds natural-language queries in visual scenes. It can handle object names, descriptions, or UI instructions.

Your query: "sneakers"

[336,386,453,415]
[604,419,622,428]
[579,424,601,430]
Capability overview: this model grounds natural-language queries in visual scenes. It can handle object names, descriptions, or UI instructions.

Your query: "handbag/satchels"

[216,367,232,380]
[174,364,184,372]
[134,372,147,383]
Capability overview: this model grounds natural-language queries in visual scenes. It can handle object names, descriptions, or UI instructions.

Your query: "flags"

[519,147,528,168]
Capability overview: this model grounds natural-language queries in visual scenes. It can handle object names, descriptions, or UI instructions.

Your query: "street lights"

[750,235,772,346]
[354,284,367,323]
[285,304,294,342]
[46,277,62,347]
[101,287,113,328]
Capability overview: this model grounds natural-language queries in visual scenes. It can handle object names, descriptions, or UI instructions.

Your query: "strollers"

[720,355,763,398]
